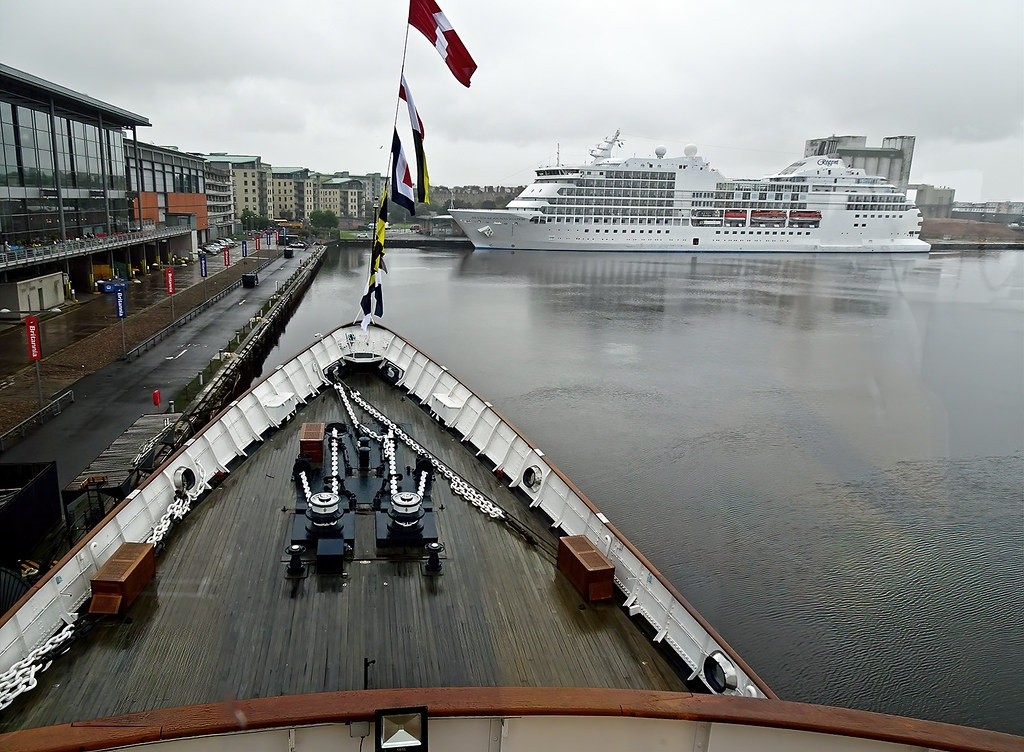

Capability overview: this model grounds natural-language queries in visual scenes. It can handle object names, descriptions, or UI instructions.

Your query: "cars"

[357,233,368,238]
[199,237,236,257]
[290,241,310,248]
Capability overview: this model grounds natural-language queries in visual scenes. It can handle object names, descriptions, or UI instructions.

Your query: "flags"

[390,126,416,217]
[398,73,432,206]
[408,0,478,88]
[358,188,389,332]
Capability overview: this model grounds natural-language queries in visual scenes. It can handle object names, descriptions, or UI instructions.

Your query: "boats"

[751,211,787,224]
[789,210,821,225]
[725,211,747,221]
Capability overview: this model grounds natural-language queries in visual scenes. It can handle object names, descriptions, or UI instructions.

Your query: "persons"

[3,231,97,261]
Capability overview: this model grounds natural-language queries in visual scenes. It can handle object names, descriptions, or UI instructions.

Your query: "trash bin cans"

[284,248,293,258]
[242,274,256,289]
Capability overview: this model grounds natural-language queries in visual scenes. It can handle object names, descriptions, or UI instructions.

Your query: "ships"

[446,127,932,253]
